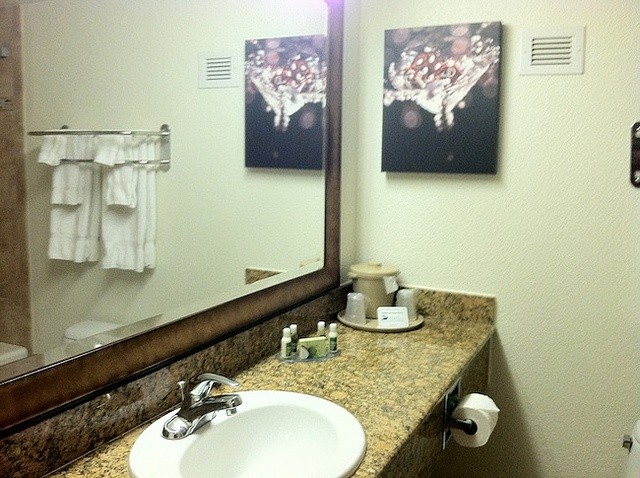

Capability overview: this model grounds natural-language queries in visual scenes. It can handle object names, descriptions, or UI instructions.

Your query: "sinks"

[128,389,367,477]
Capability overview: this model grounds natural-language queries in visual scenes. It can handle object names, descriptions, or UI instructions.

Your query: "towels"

[36,129,161,275]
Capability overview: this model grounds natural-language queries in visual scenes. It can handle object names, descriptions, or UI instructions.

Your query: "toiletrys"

[316,321,325,337]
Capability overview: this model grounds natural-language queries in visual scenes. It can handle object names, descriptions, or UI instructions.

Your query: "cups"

[344,292,367,325]
[396,289,417,322]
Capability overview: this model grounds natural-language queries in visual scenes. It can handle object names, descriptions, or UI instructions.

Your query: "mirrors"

[0,0,345,441]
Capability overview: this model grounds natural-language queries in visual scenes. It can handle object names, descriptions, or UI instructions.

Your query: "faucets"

[162,372,243,440]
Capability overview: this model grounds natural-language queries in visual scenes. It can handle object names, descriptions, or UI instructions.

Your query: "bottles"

[317,321,326,337]
